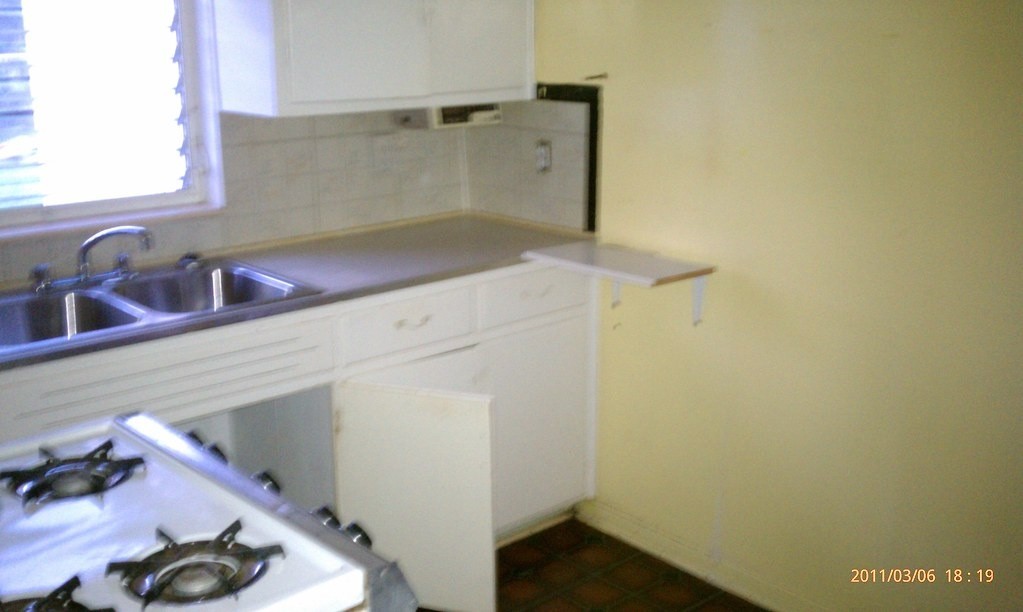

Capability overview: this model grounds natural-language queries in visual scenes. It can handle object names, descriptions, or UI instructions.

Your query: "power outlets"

[536,140,552,175]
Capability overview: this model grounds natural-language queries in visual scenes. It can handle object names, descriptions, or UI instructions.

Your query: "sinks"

[0,283,158,355]
[103,255,327,322]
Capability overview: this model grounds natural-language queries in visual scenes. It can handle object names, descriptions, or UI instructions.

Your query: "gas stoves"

[0,408,421,612]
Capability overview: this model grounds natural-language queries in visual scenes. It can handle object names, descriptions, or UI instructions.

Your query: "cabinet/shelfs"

[239,214,598,547]
[213,0,537,117]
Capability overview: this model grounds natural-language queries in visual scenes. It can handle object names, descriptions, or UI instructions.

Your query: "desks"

[519,238,716,326]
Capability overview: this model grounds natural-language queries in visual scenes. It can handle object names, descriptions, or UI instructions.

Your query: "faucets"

[74,222,155,284]
